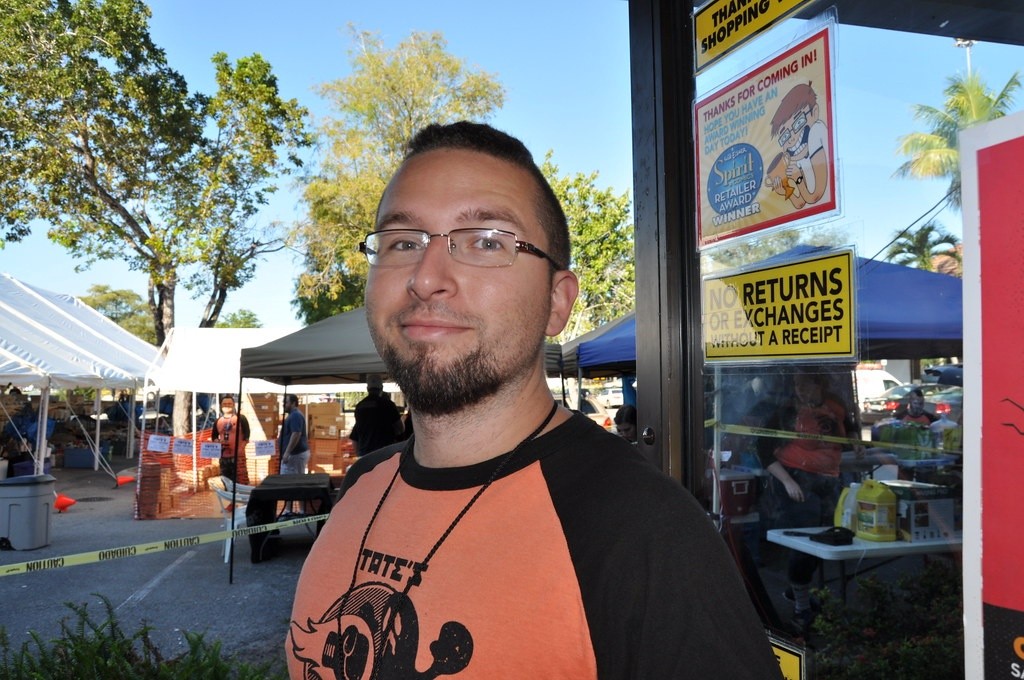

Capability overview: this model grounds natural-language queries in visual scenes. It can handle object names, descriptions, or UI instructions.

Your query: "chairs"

[221,476,255,494]
[216,488,250,564]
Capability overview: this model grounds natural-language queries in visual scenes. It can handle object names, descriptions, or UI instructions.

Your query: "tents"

[131,320,406,520]
[225,301,566,583]
[0,272,162,490]
[558,244,964,541]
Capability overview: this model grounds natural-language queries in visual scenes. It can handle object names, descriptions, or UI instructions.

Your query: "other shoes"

[781,583,820,634]
[220,503,239,513]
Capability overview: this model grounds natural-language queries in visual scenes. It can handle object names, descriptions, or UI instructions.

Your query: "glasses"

[360,227,563,273]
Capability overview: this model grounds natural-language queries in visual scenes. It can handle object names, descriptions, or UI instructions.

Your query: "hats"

[367,374,382,389]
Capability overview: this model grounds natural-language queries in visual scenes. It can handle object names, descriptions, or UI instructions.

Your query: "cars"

[907,381,963,426]
[597,388,624,410]
[865,381,950,425]
[553,395,614,430]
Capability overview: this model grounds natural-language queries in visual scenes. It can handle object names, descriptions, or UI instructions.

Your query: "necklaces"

[333,397,560,679]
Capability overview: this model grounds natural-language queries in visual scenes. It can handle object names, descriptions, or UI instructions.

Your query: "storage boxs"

[138,461,218,518]
[63,445,103,469]
[703,467,755,516]
[0,473,57,551]
[13,460,52,477]
[246,393,346,440]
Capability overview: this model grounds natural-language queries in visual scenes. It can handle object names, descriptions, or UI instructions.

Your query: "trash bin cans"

[0,475,57,551]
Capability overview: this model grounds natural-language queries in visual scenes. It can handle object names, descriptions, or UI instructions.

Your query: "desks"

[246,473,333,563]
[767,524,962,610]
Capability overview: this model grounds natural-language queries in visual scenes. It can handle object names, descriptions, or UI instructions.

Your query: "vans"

[851,369,906,424]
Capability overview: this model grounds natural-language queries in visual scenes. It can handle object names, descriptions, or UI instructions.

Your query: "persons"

[282,123,794,680]
[277,392,311,515]
[348,375,407,456]
[209,396,252,514]
